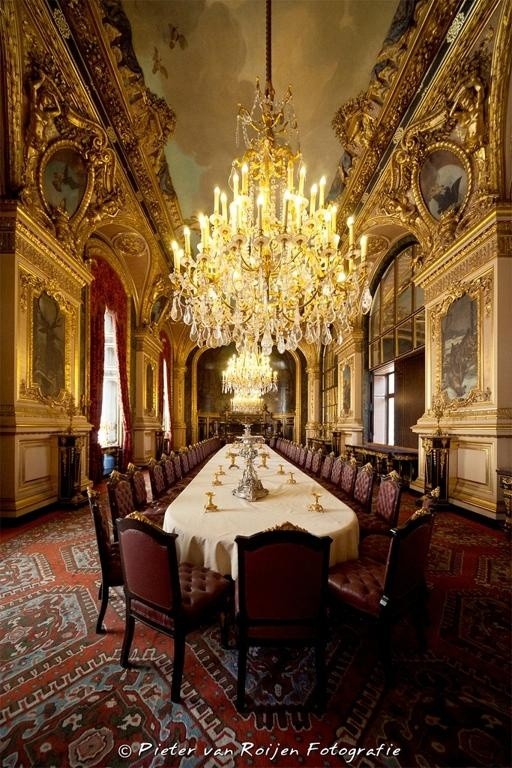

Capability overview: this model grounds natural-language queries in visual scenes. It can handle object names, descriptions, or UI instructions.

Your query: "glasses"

[63,395,77,435]
[431,394,447,434]
[220,344,279,416]
[170,0,372,359]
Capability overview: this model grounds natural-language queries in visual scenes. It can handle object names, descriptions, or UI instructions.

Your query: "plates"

[415,432,454,512]
[55,431,88,506]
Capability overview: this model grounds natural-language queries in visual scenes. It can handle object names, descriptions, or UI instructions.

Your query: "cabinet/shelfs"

[55,431,88,506]
[415,432,454,512]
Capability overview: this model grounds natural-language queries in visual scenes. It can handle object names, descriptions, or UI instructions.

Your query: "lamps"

[431,394,447,434]
[220,344,279,416]
[63,395,77,435]
[170,0,372,359]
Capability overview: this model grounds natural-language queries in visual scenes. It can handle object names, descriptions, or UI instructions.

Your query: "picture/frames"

[148,286,173,327]
[411,143,475,236]
[145,359,157,412]
[429,275,485,410]
[38,141,95,229]
[22,272,77,410]
[341,363,353,416]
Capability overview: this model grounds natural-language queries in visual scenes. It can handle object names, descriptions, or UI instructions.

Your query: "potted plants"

[38,141,95,229]
[145,359,157,412]
[429,275,485,410]
[22,272,77,410]
[411,143,475,236]
[341,363,353,416]
[148,286,173,327]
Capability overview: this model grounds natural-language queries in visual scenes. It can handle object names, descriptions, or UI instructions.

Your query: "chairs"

[86,429,437,713]
